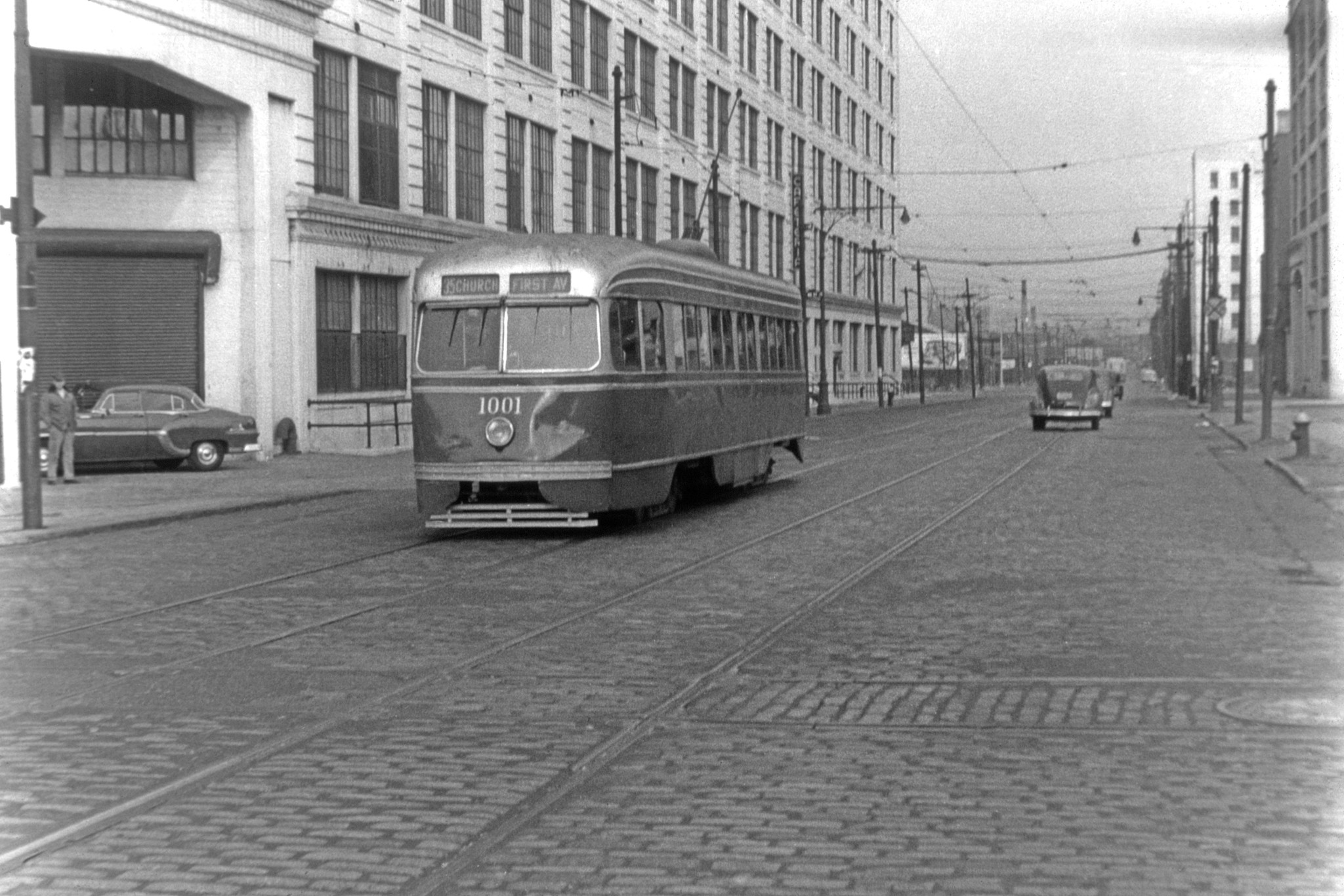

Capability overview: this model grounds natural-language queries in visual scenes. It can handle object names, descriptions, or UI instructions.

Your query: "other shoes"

[64,478,79,483]
[47,479,56,485]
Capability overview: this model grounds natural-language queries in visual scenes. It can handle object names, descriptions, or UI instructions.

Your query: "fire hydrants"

[1288,410,1312,456]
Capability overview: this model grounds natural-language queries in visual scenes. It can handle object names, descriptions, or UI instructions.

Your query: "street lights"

[817,203,913,415]
[1130,223,1223,411]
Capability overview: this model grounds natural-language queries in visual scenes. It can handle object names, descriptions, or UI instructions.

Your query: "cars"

[1027,363,1124,431]
[40,384,263,480]
[1141,369,1158,384]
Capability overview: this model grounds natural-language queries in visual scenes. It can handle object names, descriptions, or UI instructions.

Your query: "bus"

[410,230,812,531]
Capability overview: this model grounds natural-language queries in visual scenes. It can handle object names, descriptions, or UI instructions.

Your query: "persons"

[39,374,80,485]
[623,328,655,368]
